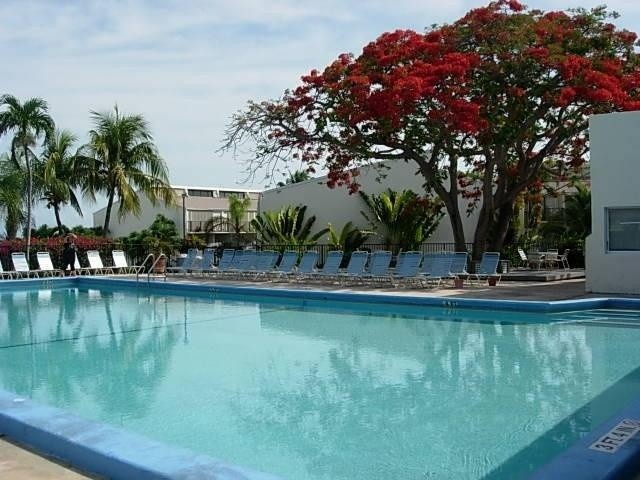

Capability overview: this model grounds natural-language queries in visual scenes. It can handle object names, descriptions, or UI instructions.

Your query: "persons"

[58,233,77,278]
[65,290,75,320]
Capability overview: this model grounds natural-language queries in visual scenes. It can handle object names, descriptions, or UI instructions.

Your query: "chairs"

[65,251,91,275]
[163,247,502,290]
[0,259,18,280]
[111,249,145,274]
[11,252,41,279]
[87,249,115,276]
[36,252,65,278]
[517,245,571,272]
